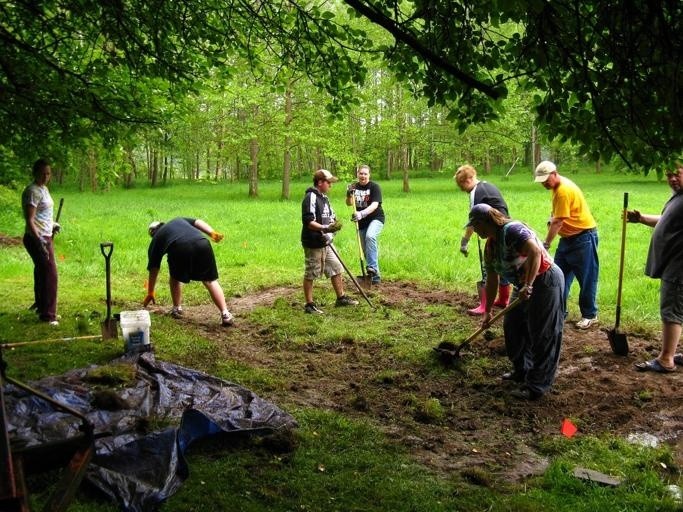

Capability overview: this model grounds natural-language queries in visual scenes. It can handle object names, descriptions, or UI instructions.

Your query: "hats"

[313,169,339,183]
[464,203,492,227]
[534,161,556,183]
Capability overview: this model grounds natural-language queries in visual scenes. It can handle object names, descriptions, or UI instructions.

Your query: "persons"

[535,161,598,329]
[23,159,62,325]
[621,168,683,374]
[346,165,385,284]
[301,170,359,315]
[455,166,512,315]
[464,204,565,399]
[144,217,233,325]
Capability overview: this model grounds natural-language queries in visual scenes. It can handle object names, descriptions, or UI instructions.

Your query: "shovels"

[432,296,522,359]
[351,188,373,292]
[477,233,489,298]
[28,197,66,312]
[100,242,119,343]
[501,157,519,182]
[607,192,629,358]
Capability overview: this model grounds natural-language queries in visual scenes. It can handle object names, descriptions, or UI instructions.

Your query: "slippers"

[635,359,677,373]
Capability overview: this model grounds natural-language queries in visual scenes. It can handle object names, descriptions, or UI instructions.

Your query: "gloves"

[209,231,224,242]
[39,236,51,261]
[347,185,355,197]
[322,222,342,245]
[460,238,468,257]
[350,209,369,222]
[144,291,155,307]
[53,222,60,235]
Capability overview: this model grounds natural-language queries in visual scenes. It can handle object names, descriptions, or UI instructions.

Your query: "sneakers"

[575,315,599,330]
[502,370,518,380]
[51,314,62,326]
[367,268,381,285]
[511,385,537,400]
[336,296,360,306]
[222,313,234,326]
[171,310,184,319]
[305,302,324,315]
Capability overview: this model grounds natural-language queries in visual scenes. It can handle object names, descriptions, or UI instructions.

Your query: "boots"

[493,284,509,309]
[467,288,487,316]
